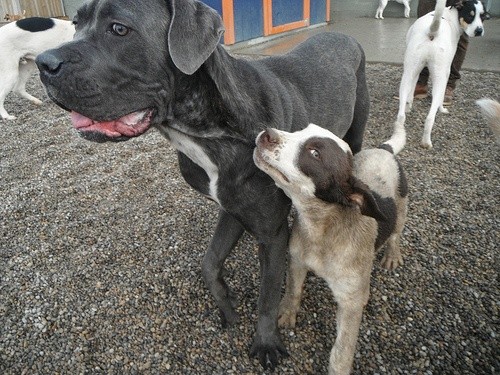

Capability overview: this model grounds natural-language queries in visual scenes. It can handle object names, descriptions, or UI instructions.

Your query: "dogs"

[36,0,369,366]
[397,1,483,147]
[0,16,78,121]
[252,124,407,375]
